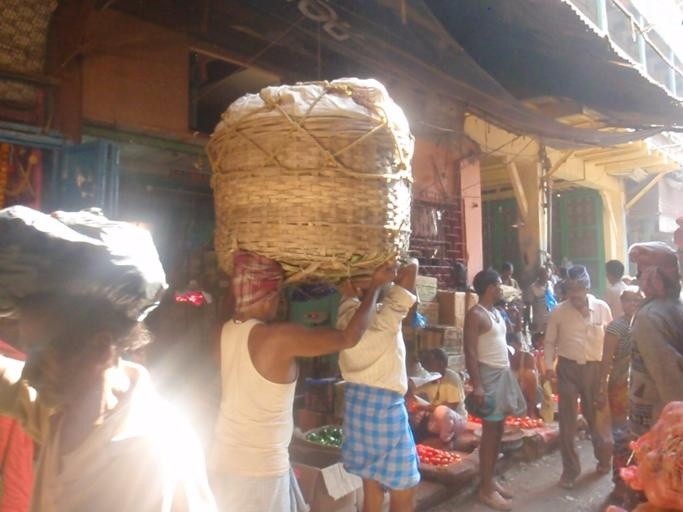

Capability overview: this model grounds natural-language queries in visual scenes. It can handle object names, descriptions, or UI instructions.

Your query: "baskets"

[204,115,414,286]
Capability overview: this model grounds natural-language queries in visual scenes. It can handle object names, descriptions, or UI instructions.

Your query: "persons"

[461,241,683,511]
[0,240,418,512]
[414,347,466,448]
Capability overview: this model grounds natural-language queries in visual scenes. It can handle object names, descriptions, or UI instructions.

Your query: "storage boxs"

[411,416,560,496]
[288,444,365,511]
